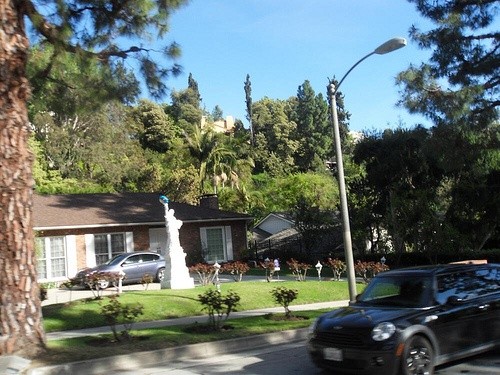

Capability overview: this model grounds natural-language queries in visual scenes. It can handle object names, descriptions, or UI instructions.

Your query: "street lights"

[158,195,170,215]
[328,37,407,306]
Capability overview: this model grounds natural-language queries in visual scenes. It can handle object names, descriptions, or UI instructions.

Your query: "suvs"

[308,262,500,375]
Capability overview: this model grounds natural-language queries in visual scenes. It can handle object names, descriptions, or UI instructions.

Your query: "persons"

[159,195,184,252]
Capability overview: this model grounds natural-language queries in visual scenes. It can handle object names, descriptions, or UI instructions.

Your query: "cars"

[77,252,166,289]
